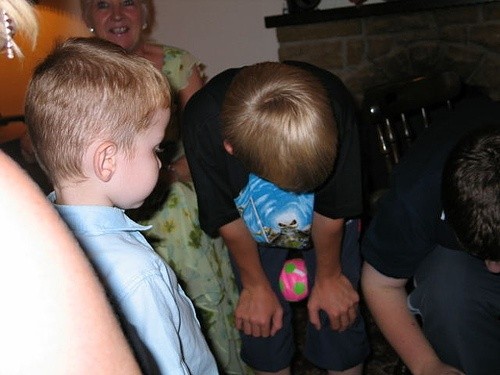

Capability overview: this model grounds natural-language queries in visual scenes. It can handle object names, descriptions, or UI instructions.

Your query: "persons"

[180,59,376,375]
[361,102,500,375]
[0,0,252,375]
